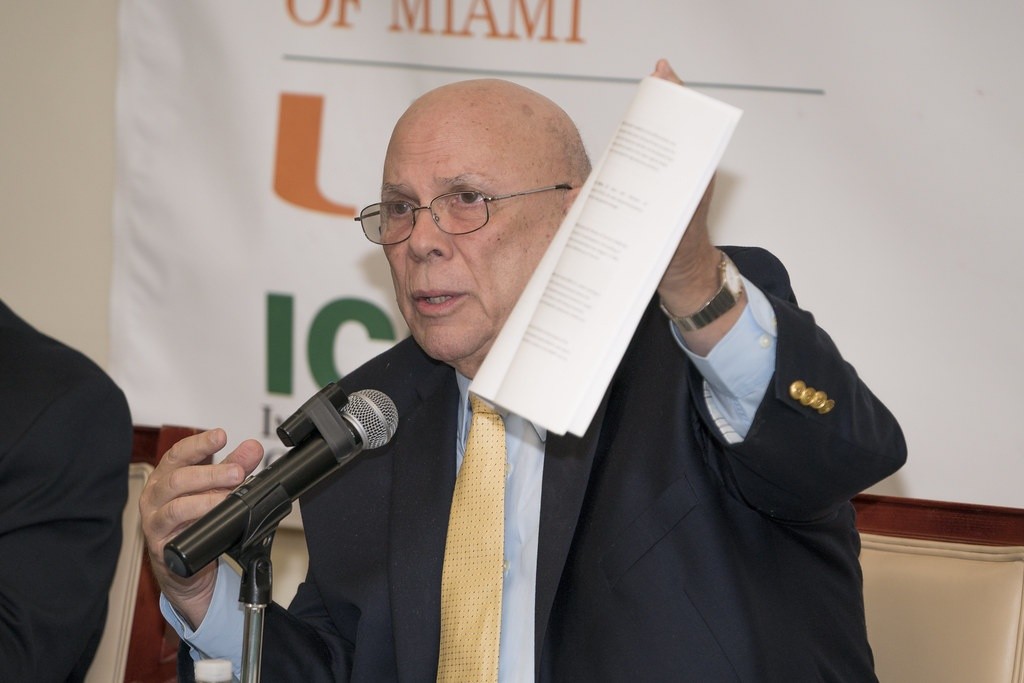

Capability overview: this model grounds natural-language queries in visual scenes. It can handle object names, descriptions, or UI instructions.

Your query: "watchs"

[657,243,746,333]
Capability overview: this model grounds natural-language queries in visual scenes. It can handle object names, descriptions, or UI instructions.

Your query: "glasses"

[354,184,575,244]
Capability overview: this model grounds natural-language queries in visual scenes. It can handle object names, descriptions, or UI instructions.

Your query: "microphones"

[163,386,402,579]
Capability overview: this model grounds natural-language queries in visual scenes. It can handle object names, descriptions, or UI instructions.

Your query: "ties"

[438,392,506,683]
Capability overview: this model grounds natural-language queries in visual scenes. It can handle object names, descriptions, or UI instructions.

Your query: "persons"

[0,299,134,683]
[135,57,907,683]
